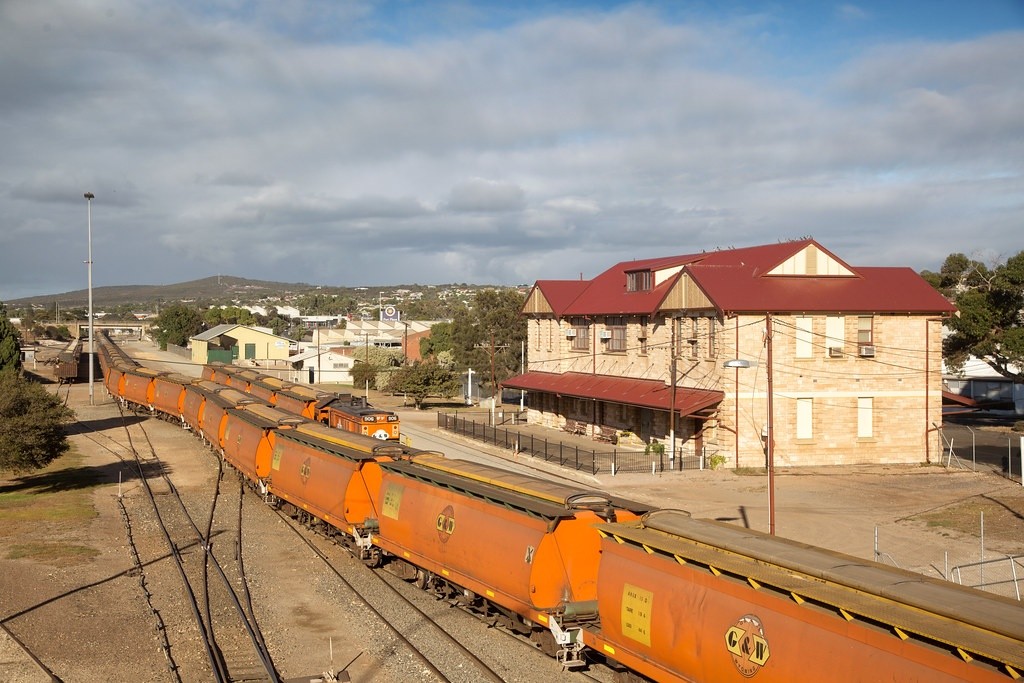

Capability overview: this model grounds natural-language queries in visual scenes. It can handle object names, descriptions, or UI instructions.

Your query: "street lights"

[82,192,96,406]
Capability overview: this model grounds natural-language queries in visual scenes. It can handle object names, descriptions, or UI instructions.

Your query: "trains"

[90,327,1024,683]
[199,361,411,449]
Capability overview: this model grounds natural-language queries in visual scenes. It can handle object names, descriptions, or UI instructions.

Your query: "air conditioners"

[687,331,698,340]
[860,346,875,356]
[600,331,611,338]
[637,330,646,338]
[565,329,576,336]
[829,346,842,356]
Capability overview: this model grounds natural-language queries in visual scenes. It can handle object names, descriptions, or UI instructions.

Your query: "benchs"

[594,424,618,444]
[563,418,576,433]
[575,421,587,435]
